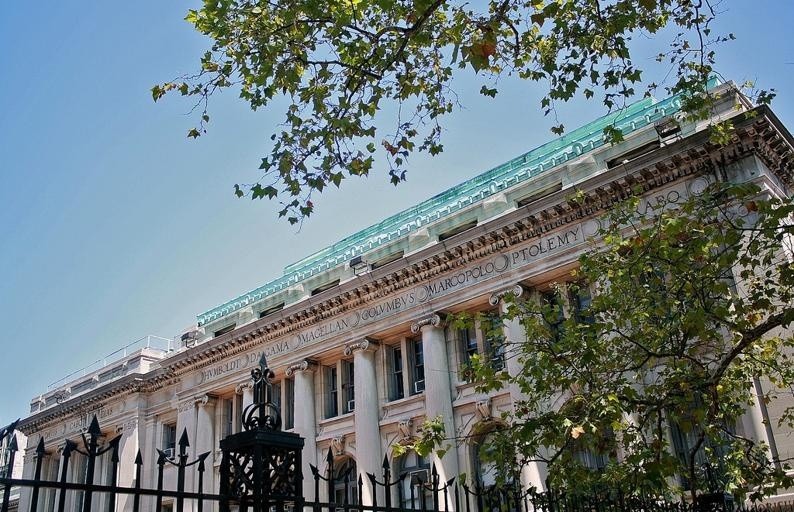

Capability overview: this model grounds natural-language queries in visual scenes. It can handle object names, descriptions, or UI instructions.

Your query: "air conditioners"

[347,400,353,413]
[410,469,429,488]
[163,448,175,460]
[415,380,425,393]
